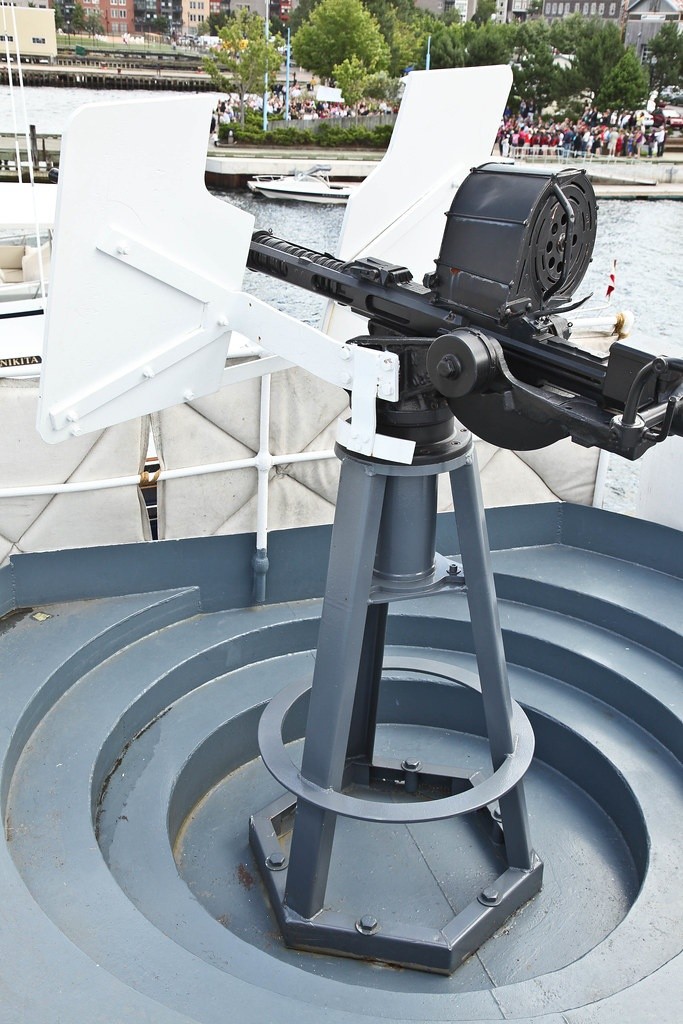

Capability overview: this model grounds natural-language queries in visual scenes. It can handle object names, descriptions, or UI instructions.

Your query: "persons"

[210,72,401,147]
[497,98,671,159]
[171,31,200,50]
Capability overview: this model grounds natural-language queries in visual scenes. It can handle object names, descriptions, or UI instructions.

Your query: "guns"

[249,155,683,466]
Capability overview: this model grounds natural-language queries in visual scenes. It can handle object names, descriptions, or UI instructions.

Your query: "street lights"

[426,36,432,71]
[282,24,290,121]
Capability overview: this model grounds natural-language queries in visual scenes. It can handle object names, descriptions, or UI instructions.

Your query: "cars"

[655,85,683,129]
[177,33,208,46]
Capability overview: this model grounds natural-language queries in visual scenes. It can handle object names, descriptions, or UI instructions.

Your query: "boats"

[247,164,350,205]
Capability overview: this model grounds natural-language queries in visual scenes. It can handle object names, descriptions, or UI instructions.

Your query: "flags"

[606,265,615,298]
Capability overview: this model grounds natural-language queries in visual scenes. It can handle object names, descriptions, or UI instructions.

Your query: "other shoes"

[607,155,611,157]
[612,155,614,157]
